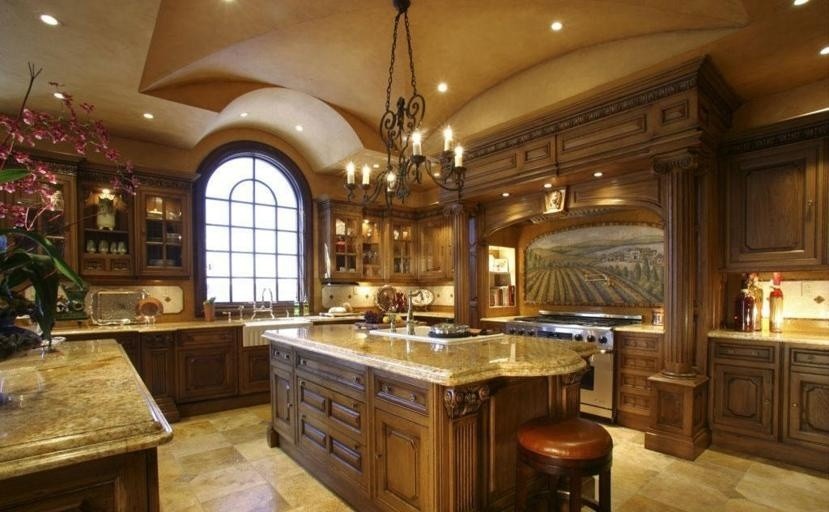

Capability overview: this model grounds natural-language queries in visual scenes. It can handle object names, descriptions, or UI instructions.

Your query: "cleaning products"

[292,295,301,317]
[302,294,310,315]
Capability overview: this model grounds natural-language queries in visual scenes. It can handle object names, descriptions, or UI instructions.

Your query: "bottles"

[490,287,503,306]
[509,285,514,304]
[752,271,764,330]
[651,306,665,326]
[303,295,309,316]
[735,271,755,331]
[293,296,302,317]
[769,273,792,335]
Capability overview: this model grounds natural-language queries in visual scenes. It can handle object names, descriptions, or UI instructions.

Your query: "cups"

[204,304,216,322]
[97,195,116,230]
[87,238,128,256]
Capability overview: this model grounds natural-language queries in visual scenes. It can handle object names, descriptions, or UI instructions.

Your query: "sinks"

[233,317,314,348]
[369,325,506,344]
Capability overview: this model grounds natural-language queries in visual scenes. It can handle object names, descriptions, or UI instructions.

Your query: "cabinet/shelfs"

[479,320,506,335]
[367,365,495,512]
[707,336,829,479]
[1,142,82,288]
[78,160,195,285]
[315,196,457,287]
[269,341,296,462]
[113,325,271,416]
[293,346,370,512]
[721,110,828,274]
[616,330,664,430]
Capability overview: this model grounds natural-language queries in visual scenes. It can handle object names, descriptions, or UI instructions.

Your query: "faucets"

[261,287,274,310]
[405,291,424,335]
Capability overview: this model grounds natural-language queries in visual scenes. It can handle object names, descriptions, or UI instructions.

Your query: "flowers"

[0,58,143,342]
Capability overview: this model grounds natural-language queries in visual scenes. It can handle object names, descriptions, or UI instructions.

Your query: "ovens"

[578,350,615,420]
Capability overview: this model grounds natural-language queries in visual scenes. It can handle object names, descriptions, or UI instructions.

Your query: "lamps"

[340,1,468,208]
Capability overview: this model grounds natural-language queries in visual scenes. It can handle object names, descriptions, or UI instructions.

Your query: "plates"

[148,233,179,243]
[135,297,164,322]
[151,259,178,265]
[40,336,66,347]
[376,285,404,312]
[410,289,435,306]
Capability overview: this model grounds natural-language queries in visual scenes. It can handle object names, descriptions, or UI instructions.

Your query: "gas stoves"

[505,313,642,352]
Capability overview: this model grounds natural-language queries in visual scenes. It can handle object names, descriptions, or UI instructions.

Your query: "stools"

[514,413,613,512]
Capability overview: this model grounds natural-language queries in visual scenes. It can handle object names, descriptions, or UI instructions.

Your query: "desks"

[0,337,174,510]
